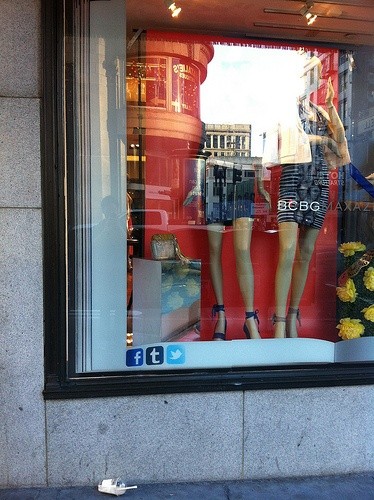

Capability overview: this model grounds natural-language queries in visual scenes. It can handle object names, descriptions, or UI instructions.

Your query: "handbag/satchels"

[151,234,191,264]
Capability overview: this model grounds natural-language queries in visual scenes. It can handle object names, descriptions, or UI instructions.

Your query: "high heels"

[242,309,261,339]
[210,304,228,341]
[272,307,302,338]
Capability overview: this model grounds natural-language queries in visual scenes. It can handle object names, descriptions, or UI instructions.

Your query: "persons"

[260,57,350,338]
[181,124,271,342]
[89,193,128,270]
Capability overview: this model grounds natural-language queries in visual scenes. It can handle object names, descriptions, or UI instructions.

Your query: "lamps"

[300,6,317,25]
[164,0,182,18]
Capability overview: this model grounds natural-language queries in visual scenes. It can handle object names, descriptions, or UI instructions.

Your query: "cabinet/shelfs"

[131,258,201,350]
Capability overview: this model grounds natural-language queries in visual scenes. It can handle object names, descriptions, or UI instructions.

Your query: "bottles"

[127,246,134,276]
[127,219,134,240]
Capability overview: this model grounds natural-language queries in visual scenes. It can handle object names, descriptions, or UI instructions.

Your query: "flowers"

[334,243,374,340]
